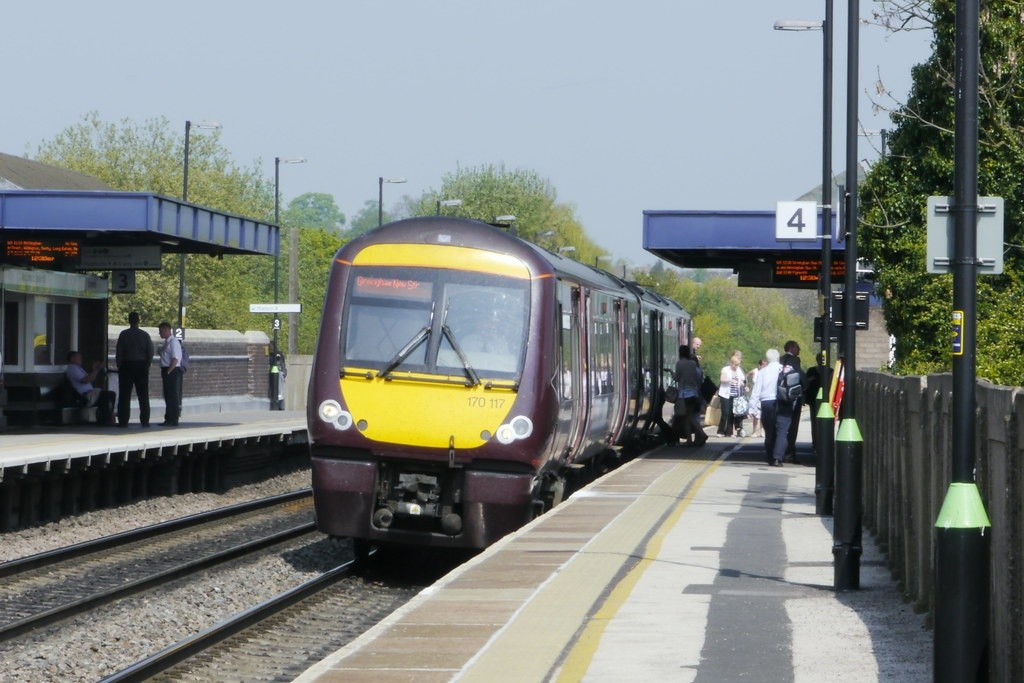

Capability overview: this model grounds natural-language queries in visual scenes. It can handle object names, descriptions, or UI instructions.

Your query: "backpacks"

[777,362,804,400]
[178,339,189,373]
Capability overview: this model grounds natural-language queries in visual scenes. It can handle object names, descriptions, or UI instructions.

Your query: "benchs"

[6,385,97,425]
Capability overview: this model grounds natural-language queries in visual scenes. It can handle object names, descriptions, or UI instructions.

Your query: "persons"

[780,343,833,457]
[269,342,286,409]
[460,314,509,354]
[158,323,182,425]
[115,311,154,426]
[748,349,793,466]
[66,351,116,425]
[668,338,709,447]
[716,351,768,436]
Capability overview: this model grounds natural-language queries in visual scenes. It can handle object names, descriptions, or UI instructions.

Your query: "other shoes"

[141,421,149,427]
[783,459,799,463]
[737,429,743,437]
[773,458,783,466]
[157,419,179,426]
[119,419,129,427]
[694,434,708,446]
[681,440,692,447]
[96,419,113,426]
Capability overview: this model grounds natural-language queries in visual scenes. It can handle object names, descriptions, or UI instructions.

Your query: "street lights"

[378,174,408,227]
[595,256,614,269]
[771,19,835,518]
[436,199,463,215]
[269,155,307,411]
[174,117,226,339]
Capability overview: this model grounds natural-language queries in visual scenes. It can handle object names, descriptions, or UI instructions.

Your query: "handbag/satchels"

[664,378,679,403]
[698,376,718,405]
[732,396,749,415]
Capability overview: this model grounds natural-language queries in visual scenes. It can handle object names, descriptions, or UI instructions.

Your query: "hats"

[67,351,83,362]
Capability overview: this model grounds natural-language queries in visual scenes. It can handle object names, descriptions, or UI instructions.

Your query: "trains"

[306,215,695,558]
